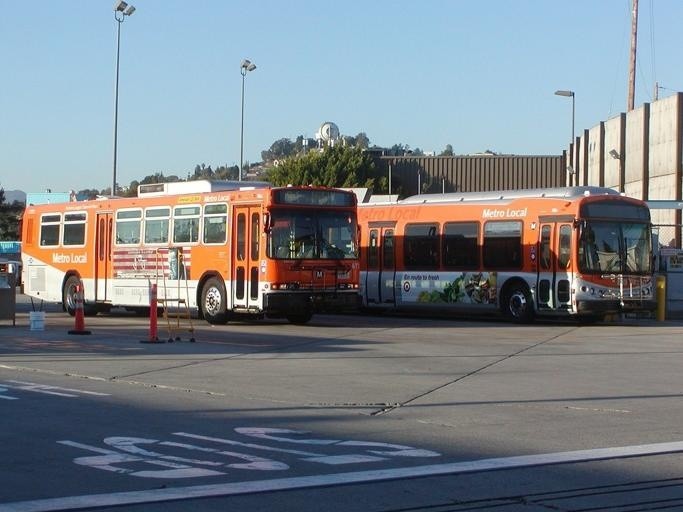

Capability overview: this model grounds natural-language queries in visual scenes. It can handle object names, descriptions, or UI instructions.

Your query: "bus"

[19,176,362,327]
[349,182,661,320]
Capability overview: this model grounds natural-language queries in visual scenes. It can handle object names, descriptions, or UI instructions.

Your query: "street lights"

[236,56,257,183]
[554,90,575,144]
[109,0,137,194]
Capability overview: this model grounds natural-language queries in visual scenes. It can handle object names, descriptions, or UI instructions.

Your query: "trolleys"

[153,246,196,344]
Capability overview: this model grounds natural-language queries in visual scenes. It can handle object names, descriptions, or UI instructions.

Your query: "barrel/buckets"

[29,310,47,332]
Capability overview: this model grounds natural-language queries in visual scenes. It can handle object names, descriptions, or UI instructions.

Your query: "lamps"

[607,147,621,163]
[563,163,577,178]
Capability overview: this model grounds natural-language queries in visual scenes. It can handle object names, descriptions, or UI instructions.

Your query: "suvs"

[5,249,21,286]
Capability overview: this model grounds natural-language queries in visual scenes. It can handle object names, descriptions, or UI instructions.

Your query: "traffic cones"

[66,278,93,335]
